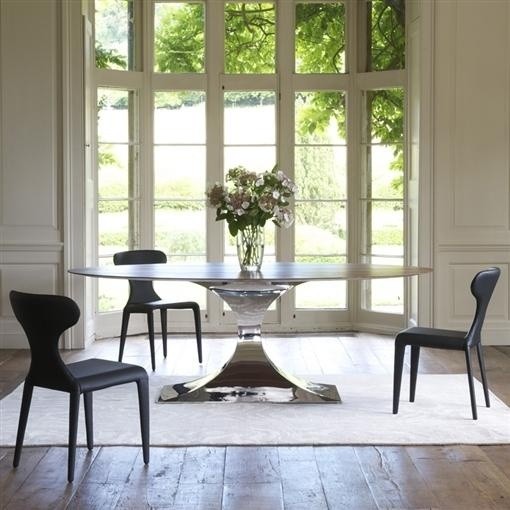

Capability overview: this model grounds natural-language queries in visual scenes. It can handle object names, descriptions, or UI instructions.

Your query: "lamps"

[8,288,150,484]
[392,266,501,421]
[113,249,202,372]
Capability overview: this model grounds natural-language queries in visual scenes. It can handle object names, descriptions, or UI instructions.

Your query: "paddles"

[0,335,510,446]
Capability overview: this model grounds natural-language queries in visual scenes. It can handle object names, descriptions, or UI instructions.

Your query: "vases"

[204,159,299,266]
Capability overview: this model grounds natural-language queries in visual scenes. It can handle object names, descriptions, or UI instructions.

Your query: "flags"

[236,225,266,271]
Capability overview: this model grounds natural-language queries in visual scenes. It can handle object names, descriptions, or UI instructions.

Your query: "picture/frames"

[65,263,434,404]
